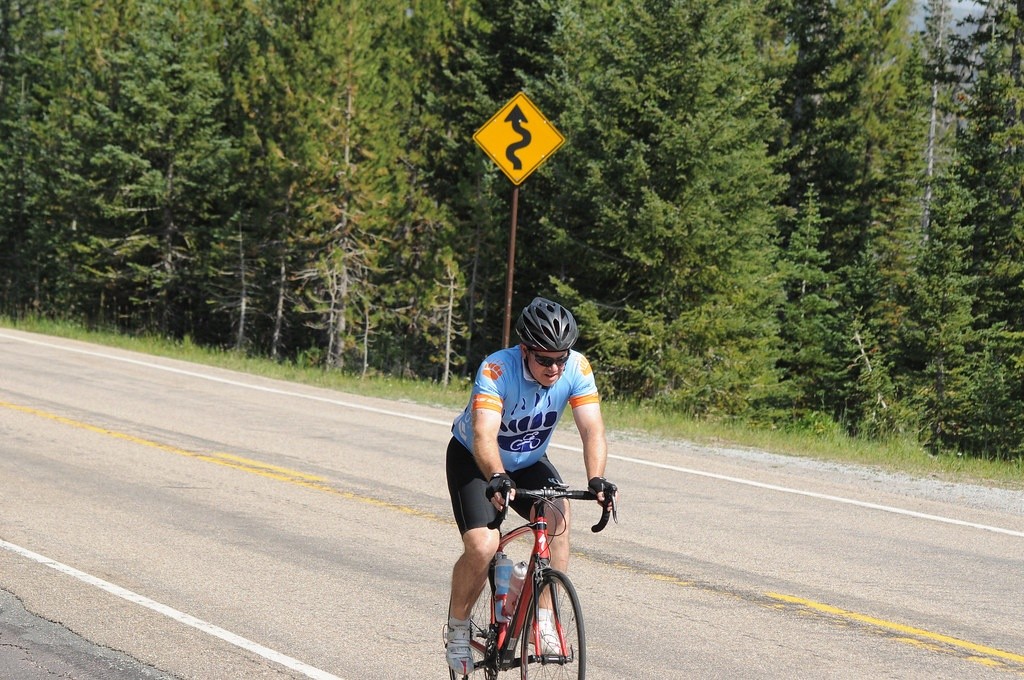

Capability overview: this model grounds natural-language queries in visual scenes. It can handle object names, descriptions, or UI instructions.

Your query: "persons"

[444,297,614,675]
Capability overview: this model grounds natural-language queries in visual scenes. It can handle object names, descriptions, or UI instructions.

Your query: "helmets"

[516,297,580,352]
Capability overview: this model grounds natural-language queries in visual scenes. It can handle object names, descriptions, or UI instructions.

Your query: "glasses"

[527,350,569,367]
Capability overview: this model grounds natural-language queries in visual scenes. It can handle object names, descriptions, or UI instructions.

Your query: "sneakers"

[528,620,562,655]
[446,615,474,675]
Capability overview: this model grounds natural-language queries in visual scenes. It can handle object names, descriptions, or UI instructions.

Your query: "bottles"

[495,554,514,622]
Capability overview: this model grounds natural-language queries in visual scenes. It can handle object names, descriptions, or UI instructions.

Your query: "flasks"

[504,560,529,615]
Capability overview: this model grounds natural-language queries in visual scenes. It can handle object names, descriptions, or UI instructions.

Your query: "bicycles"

[444,480,619,680]
